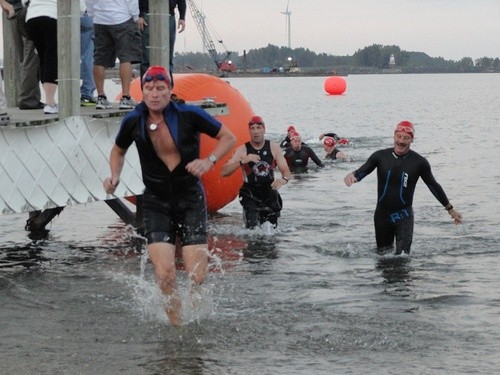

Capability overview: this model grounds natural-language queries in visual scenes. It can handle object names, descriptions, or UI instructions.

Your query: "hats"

[251,116,265,128]
[324,138,334,147]
[287,126,295,132]
[394,121,414,139]
[142,65,171,88]
[339,139,348,144]
[292,133,299,137]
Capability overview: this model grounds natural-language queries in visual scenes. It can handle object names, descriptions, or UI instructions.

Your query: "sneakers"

[80,96,97,106]
[43,103,58,113]
[95,97,113,109]
[19,101,45,109]
[118,96,137,109]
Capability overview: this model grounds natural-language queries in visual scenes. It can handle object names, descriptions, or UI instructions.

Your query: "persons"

[101,64,238,330]
[21,0,61,115]
[136,0,187,104]
[220,114,294,230]
[78,0,100,109]
[323,137,346,164]
[344,121,466,260]
[278,125,325,174]
[0,0,47,111]
[84,0,140,111]
[318,132,349,148]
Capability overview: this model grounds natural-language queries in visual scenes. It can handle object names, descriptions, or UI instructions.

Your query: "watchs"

[208,154,217,164]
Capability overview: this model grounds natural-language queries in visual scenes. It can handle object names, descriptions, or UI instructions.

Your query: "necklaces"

[148,123,165,132]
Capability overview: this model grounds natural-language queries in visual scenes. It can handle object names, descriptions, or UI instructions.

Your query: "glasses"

[248,121,264,127]
[395,125,414,134]
[142,74,169,84]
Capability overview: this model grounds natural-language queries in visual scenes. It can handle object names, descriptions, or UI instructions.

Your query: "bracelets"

[445,204,454,211]
[239,157,244,167]
[283,176,290,182]
